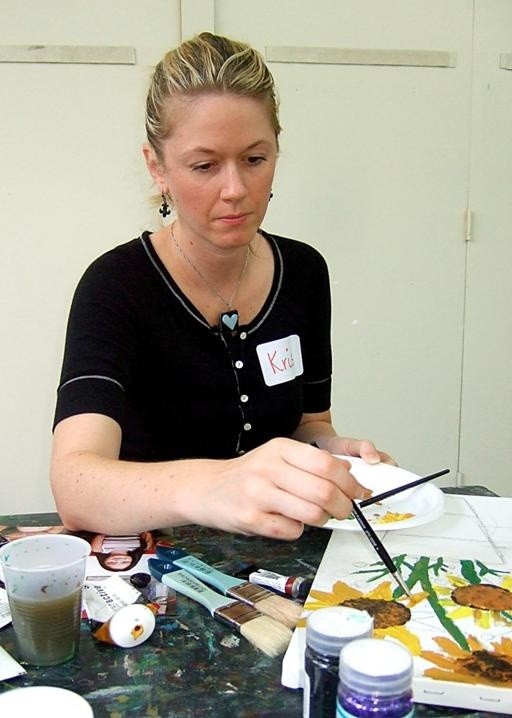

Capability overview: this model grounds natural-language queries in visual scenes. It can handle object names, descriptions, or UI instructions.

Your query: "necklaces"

[166,213,256,330]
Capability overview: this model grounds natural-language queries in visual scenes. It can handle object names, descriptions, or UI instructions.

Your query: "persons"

[87,530,154,572]
[44,28,401,542]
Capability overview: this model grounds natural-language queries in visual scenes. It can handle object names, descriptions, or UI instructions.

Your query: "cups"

[0,533,93,666]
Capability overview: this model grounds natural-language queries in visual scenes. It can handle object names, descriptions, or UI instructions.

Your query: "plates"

[303,453,445,534]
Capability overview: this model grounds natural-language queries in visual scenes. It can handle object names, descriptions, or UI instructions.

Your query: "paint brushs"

[357,469,450,510]
[310,441,417,602]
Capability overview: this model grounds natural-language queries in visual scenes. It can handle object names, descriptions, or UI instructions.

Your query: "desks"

[0,485,511,715]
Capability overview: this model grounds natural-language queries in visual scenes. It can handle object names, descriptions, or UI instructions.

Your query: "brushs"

[147,558,294,660]
[154,545,303,629]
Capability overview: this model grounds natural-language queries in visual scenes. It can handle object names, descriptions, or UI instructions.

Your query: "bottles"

[331,636,419,717]
[302,606,374,716]
